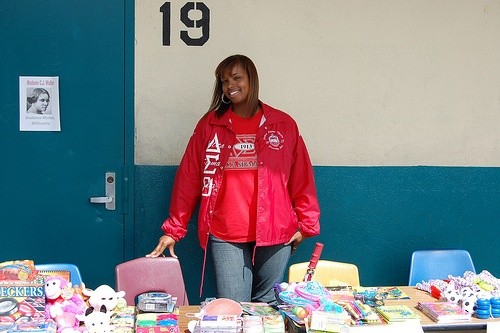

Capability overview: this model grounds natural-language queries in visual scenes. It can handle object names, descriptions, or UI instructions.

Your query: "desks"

[176,284,500,333]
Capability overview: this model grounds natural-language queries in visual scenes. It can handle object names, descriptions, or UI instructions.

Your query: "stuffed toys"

[45,275,127,333]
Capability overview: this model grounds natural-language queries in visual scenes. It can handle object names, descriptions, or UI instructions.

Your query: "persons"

[27,87,50,116]
[145,54,321,306]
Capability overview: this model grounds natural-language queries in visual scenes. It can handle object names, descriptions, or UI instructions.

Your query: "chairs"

[408,249,476,286]
[34,263,82,289]
[288,260,360,287]
[115,256,189,307]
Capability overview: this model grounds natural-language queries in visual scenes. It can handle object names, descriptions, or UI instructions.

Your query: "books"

[382,286,412,301]
[415,301,471,325]
[375,303,423,326]
